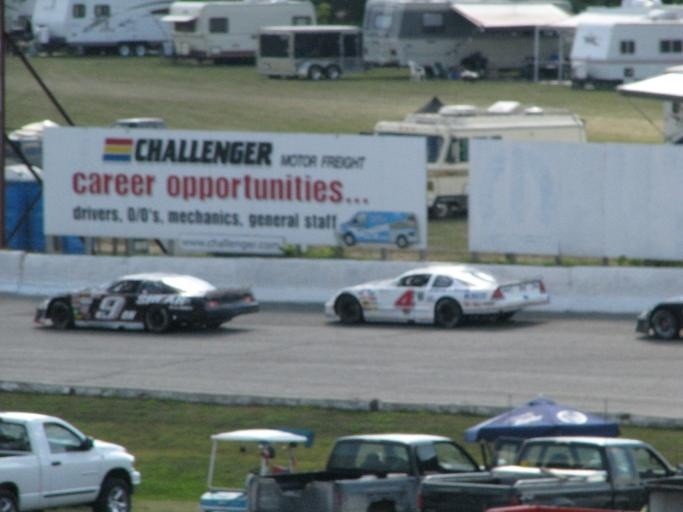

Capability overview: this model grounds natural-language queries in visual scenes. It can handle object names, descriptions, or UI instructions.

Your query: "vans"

[340,211,421,249]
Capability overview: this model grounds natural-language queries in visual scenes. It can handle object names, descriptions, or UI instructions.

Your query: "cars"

[635,294,683,340]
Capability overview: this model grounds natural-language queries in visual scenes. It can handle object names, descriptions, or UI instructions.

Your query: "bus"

[374,101,588,219]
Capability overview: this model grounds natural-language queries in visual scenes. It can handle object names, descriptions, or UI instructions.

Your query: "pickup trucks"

[0,411,141,511]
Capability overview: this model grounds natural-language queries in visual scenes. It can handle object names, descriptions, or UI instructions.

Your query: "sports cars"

[34,272,258,334]
[325,265,549,329]
